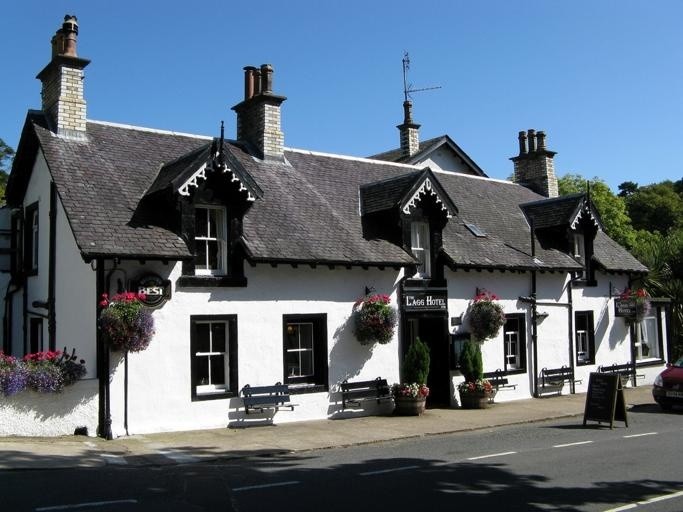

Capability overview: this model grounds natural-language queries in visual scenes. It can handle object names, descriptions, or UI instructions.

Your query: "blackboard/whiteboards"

[615,374,626,420]
[585,372,619,422]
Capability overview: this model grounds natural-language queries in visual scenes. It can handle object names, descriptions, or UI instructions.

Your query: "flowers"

[618,282,653,325]
[0,346,88,399]
[350,285,398,346]
[458,378,493,391]
[96,290,158,354]
[394,381,429,396]
[466,286,508,343]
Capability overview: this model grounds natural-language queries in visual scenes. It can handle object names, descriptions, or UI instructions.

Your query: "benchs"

[539,365,582,394]
[240,382,300,417]
[337,375,393,407]
[597,362,646,388]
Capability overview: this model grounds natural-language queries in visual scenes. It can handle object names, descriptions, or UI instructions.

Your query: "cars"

[652,354,683,410]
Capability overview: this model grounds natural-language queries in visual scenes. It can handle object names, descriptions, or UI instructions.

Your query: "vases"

[394,396,426,417]
[459,391,491,409]
